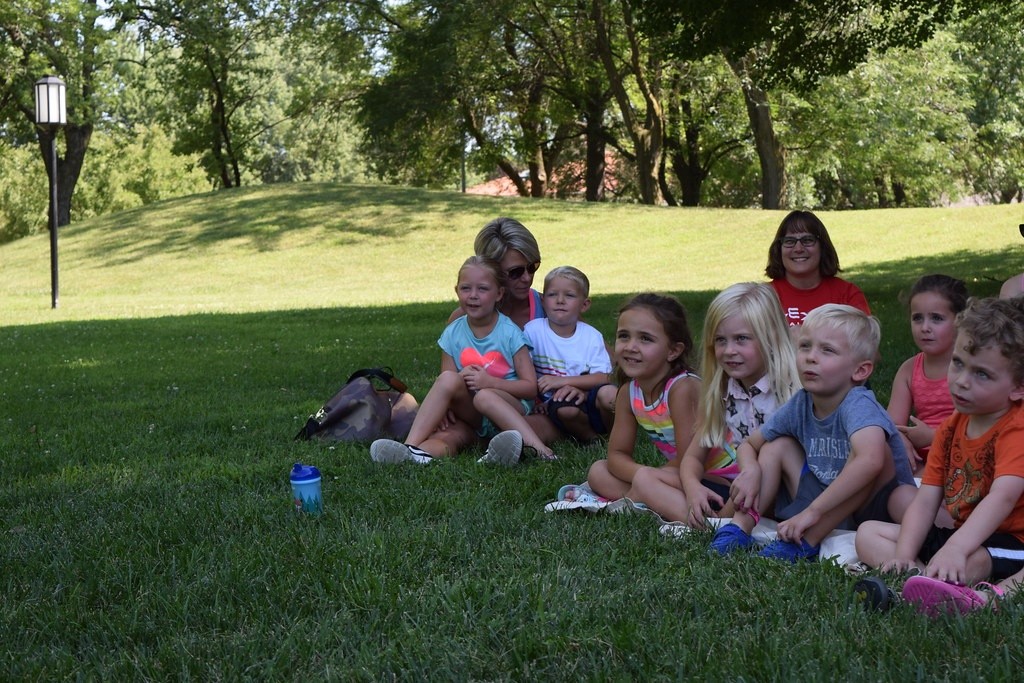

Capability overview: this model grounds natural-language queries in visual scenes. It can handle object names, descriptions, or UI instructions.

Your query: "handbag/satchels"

[291,366,421,444]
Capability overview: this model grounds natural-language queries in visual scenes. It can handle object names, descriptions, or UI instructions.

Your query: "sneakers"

[752,537,821,567]
[477,430,528,472]
[369,438,436,469]
[903,575,1005,625]
[704,522,756,555]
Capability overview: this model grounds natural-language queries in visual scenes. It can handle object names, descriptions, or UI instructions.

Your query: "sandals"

[556,484,612,507]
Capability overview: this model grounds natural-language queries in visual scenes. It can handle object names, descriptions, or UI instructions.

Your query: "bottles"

[290,463,322,514]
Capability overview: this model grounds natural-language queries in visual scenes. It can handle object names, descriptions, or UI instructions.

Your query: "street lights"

[33,73,67,309]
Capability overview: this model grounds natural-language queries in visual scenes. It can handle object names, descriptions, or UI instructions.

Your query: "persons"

[401,255,558,462]
[901,566,1024,618]
[707,303,918,566]
[887,274,970,490]
[587,294,741,506]
[521,267,620,445]
[632,281,804,530]
[999,273,1024,302]
[850,295,1024,615]
[766,210,872,332]
[369,217,615,468]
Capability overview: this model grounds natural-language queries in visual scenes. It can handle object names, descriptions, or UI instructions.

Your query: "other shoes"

[849,574,903,619]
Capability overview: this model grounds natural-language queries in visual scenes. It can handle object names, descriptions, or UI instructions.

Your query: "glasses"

[500,259,541,280]
[778,234,821,248]
